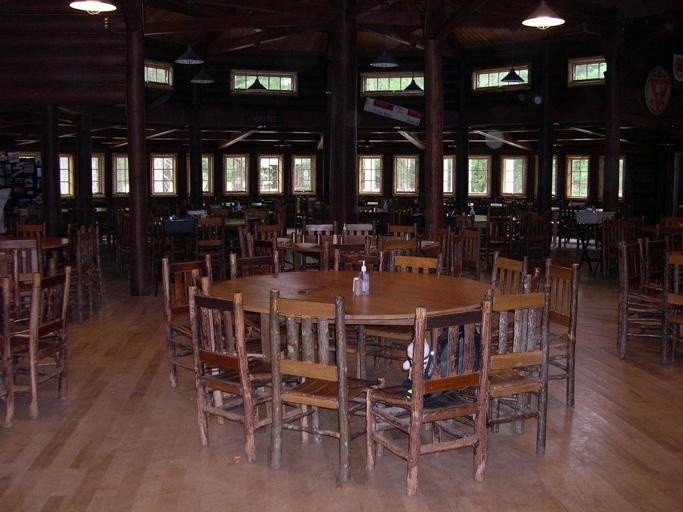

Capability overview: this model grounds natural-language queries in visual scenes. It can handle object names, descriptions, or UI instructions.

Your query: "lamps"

[370,34,400,66]
[175,41,204,64]
[191,65,215,83]
[404,46,423,92]
[522,0,566,30]
[249,45,267,90]
[69,0,117,15]
[502,55,524,83]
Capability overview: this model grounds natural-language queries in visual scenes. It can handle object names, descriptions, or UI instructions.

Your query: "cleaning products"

[357,260,370,296]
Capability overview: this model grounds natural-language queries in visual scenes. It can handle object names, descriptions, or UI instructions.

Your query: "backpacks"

[438,326,482,377]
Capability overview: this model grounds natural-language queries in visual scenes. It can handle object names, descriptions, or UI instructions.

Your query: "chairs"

[195,212,225,281]
[159,251,215,391]
[61,225,94,319]
[395,256,441,275]
[522,212,552,281]
[638,237,663,331]
[270,289,388,480]
[482,218,513,270]
[474,201,491,214]
[476,258,525,344]
[340,255,377,271]
[0,279,16,427]
[425,225,457,275]
[600,222,627,279]
[525,259,582,414]
[387,222,418,240]
[17,219,47,266]
[277,205,287,236]
[258,224,282,240]
[377,235,421,273]
[239,225,272,275]
[342,221,375,235]
[618,241,663,359]
[559,205,574,247]
[432,285,553,457]
[11,265,73,419]
[227,250,287,279]
[664,249,683,363]
[183,286,258,467]
[150,219,165,247]
[389,196,416,222]
[243,209,256,230]
[358,206,377,226]
[501,201,514,217]
[294,219,336,271]
[367,301,491,494]
[457,214,475,231]
[0,241,38,307]
[248,230,284,275]
[256,211,269,224]
[331,233,371,271]
[667,218,683,226]
[450,226,484,283]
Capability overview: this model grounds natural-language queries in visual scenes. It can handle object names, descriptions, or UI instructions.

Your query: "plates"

[295,243,317,250]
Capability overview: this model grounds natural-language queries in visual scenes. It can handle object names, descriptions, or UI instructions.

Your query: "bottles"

[470,207,475,216]
[383,201,388,210]
[355,280,361,296]
[353,277,359,293]
[333,221,338,244]
[359,261,369,296]
[340,236,344,245]
[342,224,347,237]
